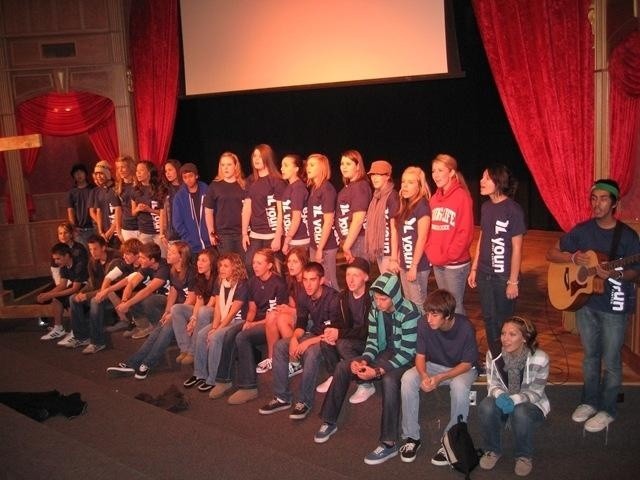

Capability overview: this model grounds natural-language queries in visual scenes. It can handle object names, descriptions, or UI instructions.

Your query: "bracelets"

[616,270,623,282]
[571,252,578,265]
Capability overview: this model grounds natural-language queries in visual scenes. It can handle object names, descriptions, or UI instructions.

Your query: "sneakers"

[572,404,595,422]
[515,457,532,476]
[41,324,105,353]
[177,353,193,365]
[184,376,258,406]
[480,451,501,469]
[349,384,375,404]
[401,437,421,462]
[259,398,291,414]
[431,448,449,466]
[289,400,310,420]
[315,422,337,444]
[363,442,398,466]
[317,376,332,394]
[289,362,303,378]
[108,320,153,339]
[585,412,614,432]
[256,359,272,373]
[106,362,151,380]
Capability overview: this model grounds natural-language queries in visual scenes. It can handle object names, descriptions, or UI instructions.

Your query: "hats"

[339,256,369,276]
[180,163,198,178]
[368,160,391,177]
[94,160,113,183]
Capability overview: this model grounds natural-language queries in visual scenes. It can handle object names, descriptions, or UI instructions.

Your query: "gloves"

[496,393,514,415]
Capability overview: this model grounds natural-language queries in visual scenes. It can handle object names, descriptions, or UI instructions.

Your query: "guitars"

[548,251,639,313]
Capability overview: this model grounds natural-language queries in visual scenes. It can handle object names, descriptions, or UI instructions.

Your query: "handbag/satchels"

[441,414,479,475]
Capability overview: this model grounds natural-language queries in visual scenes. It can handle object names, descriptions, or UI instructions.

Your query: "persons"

[477,314,551,476]
[38,144,528,467]
[544,178,640,434]
